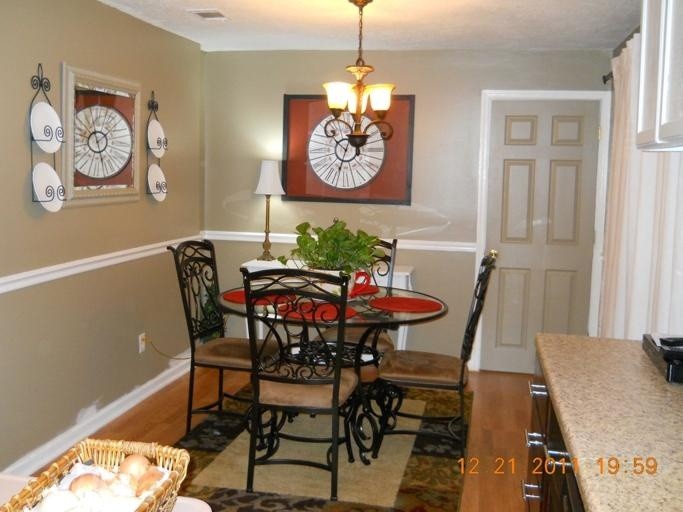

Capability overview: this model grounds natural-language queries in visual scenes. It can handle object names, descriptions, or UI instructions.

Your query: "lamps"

[321,5,396,150]
[248,157,285,263]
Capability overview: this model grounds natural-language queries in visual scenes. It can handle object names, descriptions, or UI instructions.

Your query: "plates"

[33,162,64,212]
[147,164,168,202]
[147,120,166,157]
[30,102,63,154]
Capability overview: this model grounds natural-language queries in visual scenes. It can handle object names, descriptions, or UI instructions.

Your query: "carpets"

[173,381,476,511]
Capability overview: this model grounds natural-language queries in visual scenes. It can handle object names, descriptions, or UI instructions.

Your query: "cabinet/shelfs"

[639,0,683,146]
[519,358,557,508]
[548,458,587,512]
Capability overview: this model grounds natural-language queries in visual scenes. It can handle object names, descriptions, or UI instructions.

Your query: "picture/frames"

[279,95,414,207]
[58,63,146,208]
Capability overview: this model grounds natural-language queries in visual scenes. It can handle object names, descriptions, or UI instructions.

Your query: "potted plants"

[277,218,383,300]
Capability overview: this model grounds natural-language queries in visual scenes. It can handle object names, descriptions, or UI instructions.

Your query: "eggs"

[118,454,152,481]
[137,470,164,498]
[69,474,111,493]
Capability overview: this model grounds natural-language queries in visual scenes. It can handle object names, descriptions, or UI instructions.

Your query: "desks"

[242,255,421,353]
[226,286,449,452]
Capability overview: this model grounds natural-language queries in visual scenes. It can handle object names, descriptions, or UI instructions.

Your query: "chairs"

[291,240,402,391]
[164,241,290,444]
[365,252,499,460]
[232,266,360,502]
